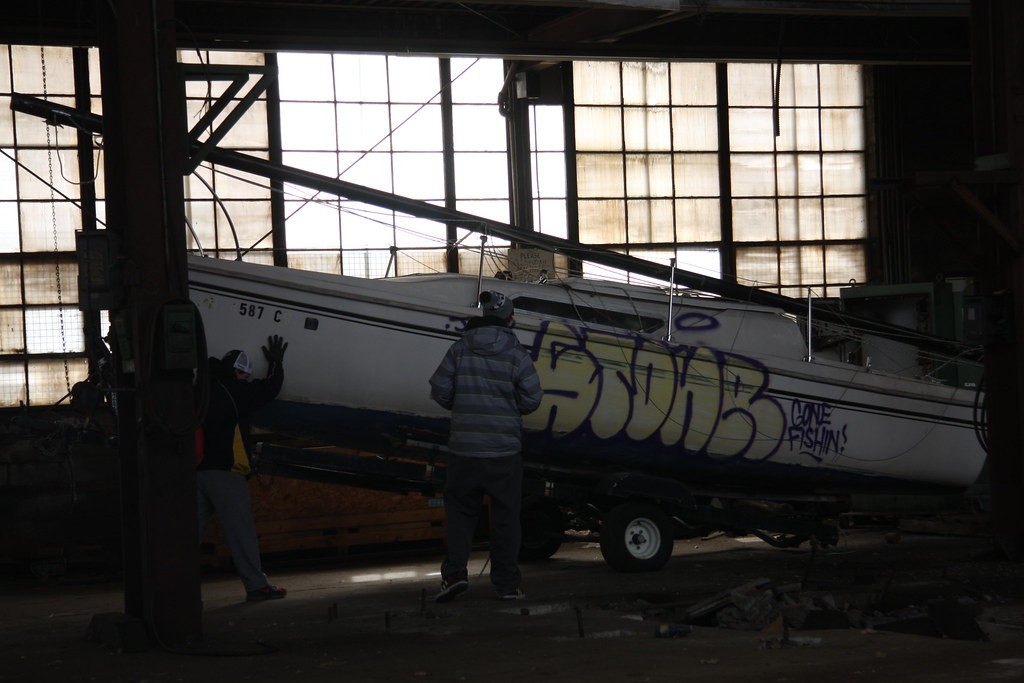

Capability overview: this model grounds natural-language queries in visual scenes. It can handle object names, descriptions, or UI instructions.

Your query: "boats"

[73,214,994,522]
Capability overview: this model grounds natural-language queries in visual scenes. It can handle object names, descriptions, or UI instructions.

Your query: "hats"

[480,292,513,320]
[221,350,252,375]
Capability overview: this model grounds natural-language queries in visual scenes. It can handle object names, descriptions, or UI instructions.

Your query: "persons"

[194,334,291,601]
[425,289,545,600]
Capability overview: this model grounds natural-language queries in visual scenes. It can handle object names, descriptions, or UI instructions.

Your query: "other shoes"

[435,571,468,602]
[247,585,287,602]
[499,587,525,599]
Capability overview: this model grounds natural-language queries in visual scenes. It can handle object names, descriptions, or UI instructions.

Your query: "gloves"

[262,335,288,367]
